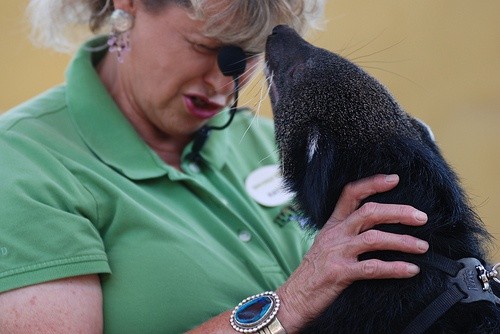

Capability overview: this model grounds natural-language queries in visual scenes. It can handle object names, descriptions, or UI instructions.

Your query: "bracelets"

[230,291,288,334]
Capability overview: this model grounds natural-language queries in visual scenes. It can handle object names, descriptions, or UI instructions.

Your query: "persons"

[0,0,428,334]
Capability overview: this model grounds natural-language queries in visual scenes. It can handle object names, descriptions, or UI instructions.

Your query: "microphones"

[203,46,247,133]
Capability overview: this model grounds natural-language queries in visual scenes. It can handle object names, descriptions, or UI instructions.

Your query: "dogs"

[211,22,500,334]
